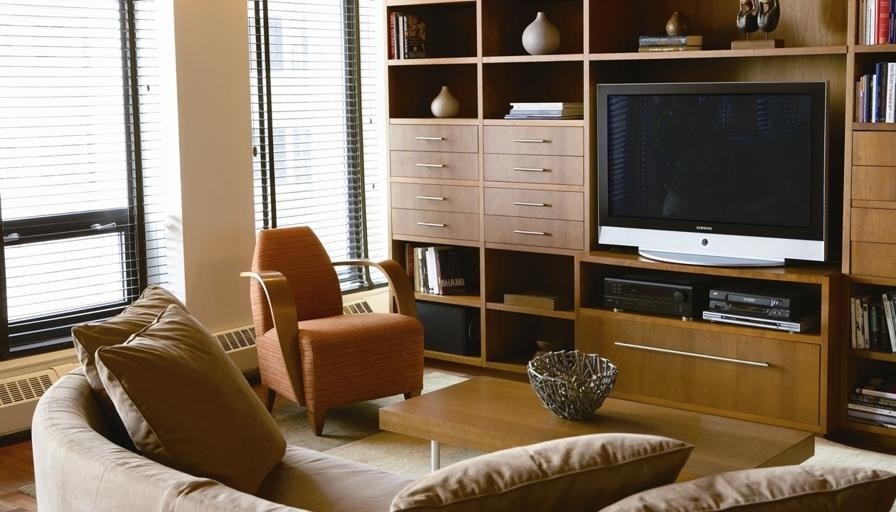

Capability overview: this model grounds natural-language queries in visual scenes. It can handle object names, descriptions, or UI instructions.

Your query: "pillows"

[70,283,197,400]
[93,302,287,495]
[389,434,696,512]
[596,464,895,512]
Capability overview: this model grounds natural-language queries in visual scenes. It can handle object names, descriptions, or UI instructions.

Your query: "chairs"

[237,225,427,438]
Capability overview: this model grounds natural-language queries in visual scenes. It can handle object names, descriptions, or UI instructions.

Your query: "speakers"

[415,301,480,356]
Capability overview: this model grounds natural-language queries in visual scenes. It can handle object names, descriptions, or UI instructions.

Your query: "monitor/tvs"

[593,81,832,268]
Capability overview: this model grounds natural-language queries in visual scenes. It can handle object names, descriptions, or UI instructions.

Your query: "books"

[389,11,433,58]
[504,102,583,120]
[851,292,896,354]
[855,62,896,124]
[859,0,896,45]
[848,377,896,429]
[405,242,467,295]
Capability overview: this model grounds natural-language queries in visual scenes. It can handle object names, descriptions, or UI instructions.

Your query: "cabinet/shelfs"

[385,0,896,438]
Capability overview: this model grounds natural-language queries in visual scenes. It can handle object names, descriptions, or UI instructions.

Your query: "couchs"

[30,357,444,512]
